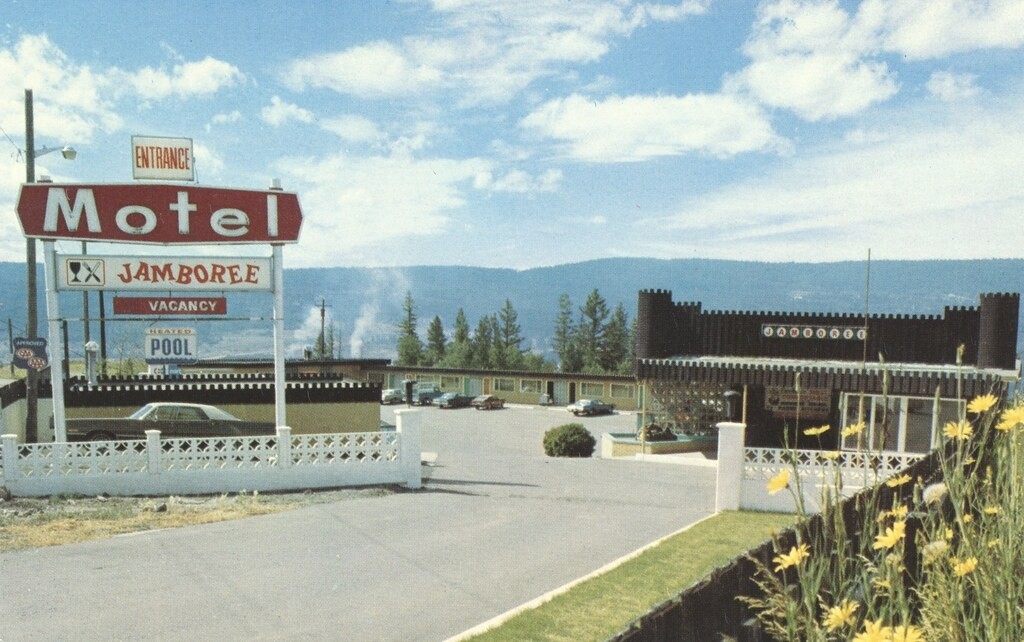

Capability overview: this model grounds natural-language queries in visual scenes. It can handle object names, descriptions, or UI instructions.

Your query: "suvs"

[410,380,445,407]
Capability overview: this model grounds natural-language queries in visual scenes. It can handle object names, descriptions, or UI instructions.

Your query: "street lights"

[24,89,77,444]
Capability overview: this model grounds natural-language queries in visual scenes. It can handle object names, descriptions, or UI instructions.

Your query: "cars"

[125,402,243,421]
[470,394,507,411]
[381,389,404,405]
[432,391,473,410]
[567,399,615,417]
